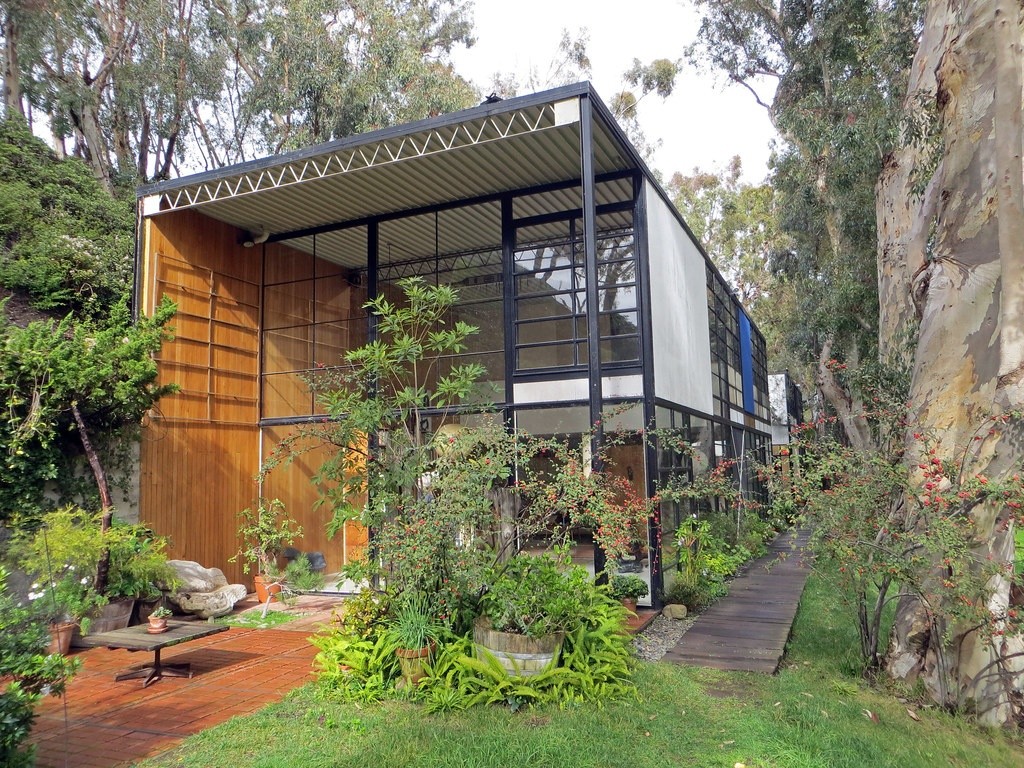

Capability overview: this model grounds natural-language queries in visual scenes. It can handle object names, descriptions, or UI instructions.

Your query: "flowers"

[17,565,108,635]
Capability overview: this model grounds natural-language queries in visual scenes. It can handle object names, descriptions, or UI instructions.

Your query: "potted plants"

[0,292,182,648]
[608,574,649,616]
[145,608,172,634]
[312,631,374,679]
[375,564,462,687]
[228,497,304,603]
[472,542,594,682]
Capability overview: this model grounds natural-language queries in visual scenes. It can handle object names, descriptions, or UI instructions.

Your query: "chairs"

[283,546,326,574]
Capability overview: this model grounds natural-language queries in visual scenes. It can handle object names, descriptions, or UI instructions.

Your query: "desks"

[82,618,231,687]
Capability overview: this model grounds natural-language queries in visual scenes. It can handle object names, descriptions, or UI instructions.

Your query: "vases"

[44,622,75,656]
